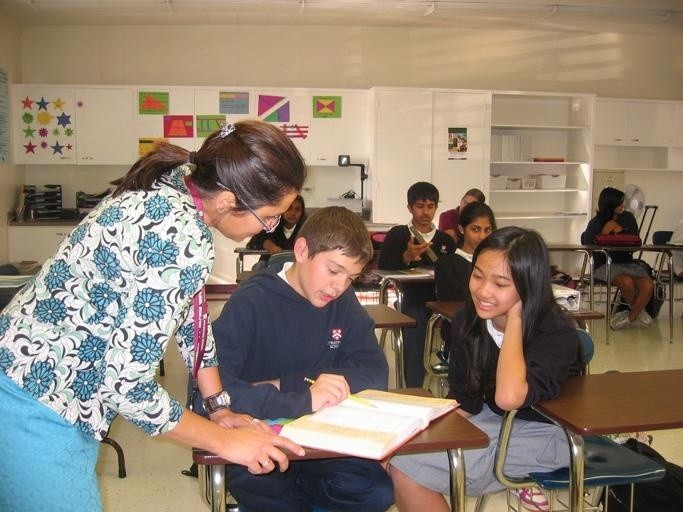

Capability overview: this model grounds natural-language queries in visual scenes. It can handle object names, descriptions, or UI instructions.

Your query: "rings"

[249,416,259,426]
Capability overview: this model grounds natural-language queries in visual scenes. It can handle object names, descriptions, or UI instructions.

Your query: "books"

[489,173,569,190]
[278,388,461,464]
[492,133,540,162]
[372,268,431,280]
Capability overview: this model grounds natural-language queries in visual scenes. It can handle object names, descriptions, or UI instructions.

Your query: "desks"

[589,234,683,345]
[364,302,418,388]
[423,299,468,396]
[541,243,593,313]
[191,387,490,512]
[234,247,296,284]
[494,367,682,512]
[377,266,436,305]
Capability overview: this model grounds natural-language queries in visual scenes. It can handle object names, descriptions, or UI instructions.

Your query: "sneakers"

[609,310,635,330]
[638,307,654,328]
[510,487,550,511]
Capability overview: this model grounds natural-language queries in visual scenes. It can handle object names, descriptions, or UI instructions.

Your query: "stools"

[526,433,666,512]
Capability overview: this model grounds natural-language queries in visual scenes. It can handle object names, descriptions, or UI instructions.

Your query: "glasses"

[216,182,280,235]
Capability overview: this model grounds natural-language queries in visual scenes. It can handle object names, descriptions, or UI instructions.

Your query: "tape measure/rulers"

[409,224,440,262]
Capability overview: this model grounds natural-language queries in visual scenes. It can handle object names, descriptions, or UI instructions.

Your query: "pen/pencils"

[303,377,382,410]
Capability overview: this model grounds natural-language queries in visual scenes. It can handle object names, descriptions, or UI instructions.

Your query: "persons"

[432,201,498,343]
[0,120,308,511]
[191,206,394,510]
[582,188,655,329]
[441,190,484,242]
[384,227,587,511]
[377,183,456,388]
[253,196,308,255]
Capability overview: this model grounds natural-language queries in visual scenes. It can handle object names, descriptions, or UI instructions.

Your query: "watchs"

[200,389,233,413]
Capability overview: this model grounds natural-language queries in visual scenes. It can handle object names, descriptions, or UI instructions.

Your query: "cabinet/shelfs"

[137,86,253,165]
[10,85,137,166]
[596,95,673,171]
[377,86,489,228]
[492,88,595,292]
[251,88,370,168]
